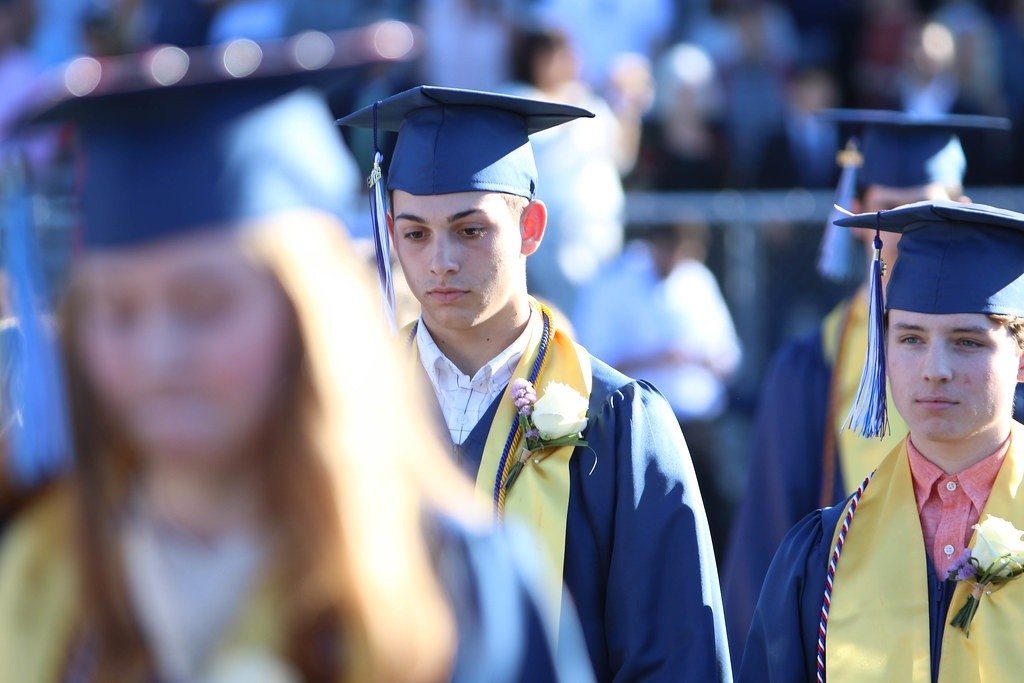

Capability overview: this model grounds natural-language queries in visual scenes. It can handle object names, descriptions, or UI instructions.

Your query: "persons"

[489,0,1024,657]
[0,55,598,683]
[730,197,1024,683]
[338,88,734,683]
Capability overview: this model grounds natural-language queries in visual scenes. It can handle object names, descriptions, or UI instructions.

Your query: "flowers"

[501,378,597,493]
[946,515,1024,639]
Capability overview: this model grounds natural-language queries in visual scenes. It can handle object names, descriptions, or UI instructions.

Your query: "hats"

[0,30,370,484]
[334,86,595,335]
[833,200,1024,442]
[815,108,1011,280]
